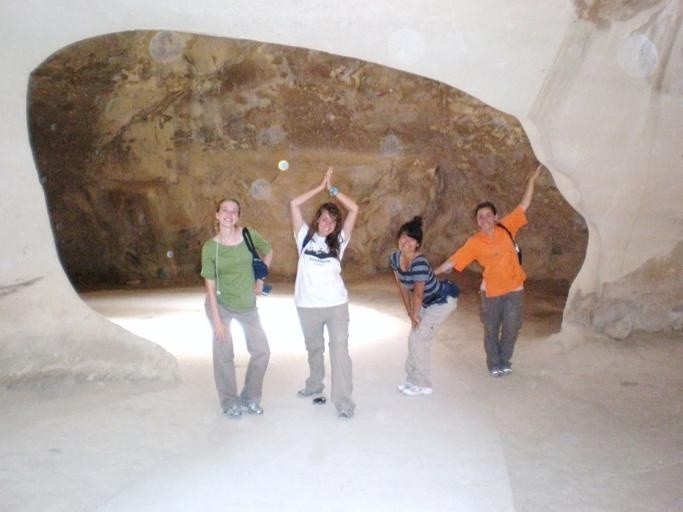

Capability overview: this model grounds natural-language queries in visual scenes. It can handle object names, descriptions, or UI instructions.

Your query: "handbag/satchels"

[242,227,269,281]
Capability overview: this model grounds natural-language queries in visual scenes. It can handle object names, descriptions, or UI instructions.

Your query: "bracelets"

[328,185,339,198]
[406,309,413,316]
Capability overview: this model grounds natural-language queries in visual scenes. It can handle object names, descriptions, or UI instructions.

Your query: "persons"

[432,163,544,375]
[389,213,460,396]
[198,196,274,420]
[288,164,359,418]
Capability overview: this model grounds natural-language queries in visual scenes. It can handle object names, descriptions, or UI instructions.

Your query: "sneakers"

[487,367,513,379]
[336,401,355,419]
[397,382,434,397]
[297,383,324,398]
[224,397,264,419]
